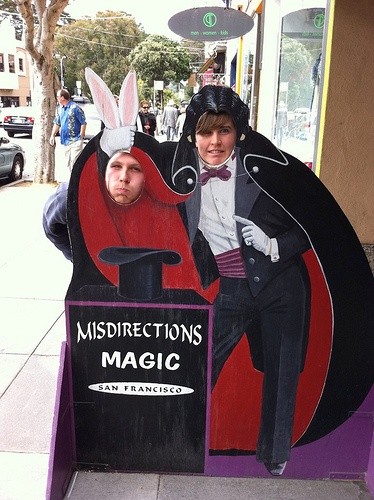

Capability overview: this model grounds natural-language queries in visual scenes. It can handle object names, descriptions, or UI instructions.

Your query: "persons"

[136,98,190,141]
[274,100,288,148]
[194,110,237,165]
[49,89,87,173]
[104,152,145,204]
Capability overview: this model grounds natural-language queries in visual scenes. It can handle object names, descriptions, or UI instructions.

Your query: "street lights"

[61,56,66,88]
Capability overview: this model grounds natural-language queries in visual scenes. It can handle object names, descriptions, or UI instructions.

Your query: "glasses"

[143,107,149,108]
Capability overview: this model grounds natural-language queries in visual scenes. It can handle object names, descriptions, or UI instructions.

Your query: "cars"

[0,136,26,182]
[3,107,60,137]
[71,95,90,104]
